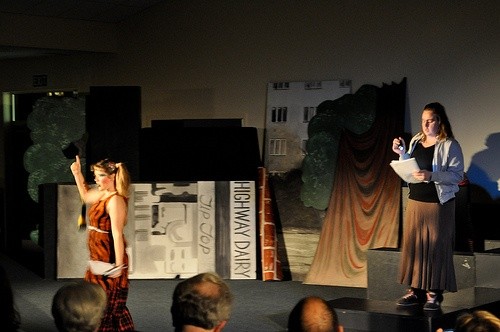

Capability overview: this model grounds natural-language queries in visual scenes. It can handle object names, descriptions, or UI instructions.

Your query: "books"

[390,157,426,184]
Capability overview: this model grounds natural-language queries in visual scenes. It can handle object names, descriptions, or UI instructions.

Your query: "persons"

[70,155,135,332]
[0,264,25,332]
[453,310,500,332]
[50,282,107,332]
[288,295,344,332]
[391,102,464,310]
[170,272,233,332]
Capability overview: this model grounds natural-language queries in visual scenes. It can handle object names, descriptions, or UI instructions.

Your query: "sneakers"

[396,289,423,306]
[423,292,442,310]
